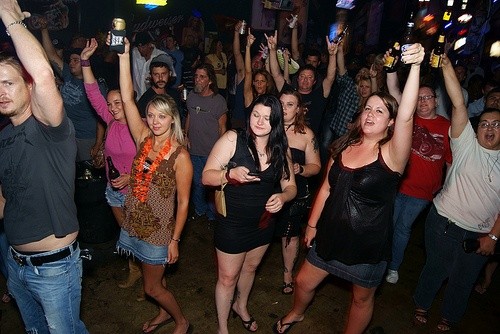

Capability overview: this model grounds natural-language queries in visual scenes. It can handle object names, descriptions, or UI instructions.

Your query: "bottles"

[431,33,446,70]
[331,26,348,43]
[383,40,400,72]
[106,156,120,191]
[401,12,415,46]
[222,63,225,75]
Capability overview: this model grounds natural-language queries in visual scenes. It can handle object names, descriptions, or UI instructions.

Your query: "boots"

[118,259,143,288]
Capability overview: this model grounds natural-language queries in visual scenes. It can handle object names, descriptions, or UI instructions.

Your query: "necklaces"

[483,147,499,184]
[131,133,172,202]
[257,151,265,157]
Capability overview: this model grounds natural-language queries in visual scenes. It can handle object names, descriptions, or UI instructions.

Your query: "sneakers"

[385,269,399,283]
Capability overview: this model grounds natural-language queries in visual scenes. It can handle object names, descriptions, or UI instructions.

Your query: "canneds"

[239,20,247,34]
[182,89,187,100]
[112,18,126,30]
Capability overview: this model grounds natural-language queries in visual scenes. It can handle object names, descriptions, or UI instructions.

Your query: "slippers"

[140,316,173,334]
[272,314,297,334]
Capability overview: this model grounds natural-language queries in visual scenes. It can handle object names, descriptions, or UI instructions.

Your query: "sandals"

[434,316,451,334]
[414,308,428,326]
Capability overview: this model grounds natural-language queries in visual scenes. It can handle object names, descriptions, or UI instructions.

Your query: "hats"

[134,32,153,47]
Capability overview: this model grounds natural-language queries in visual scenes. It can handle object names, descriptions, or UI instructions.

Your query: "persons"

[105,30,194,334]
[202,94,297,334]
[0,15,499,304]
[273,43,425,334]
[0,0,83,334]
[413,50,500,334]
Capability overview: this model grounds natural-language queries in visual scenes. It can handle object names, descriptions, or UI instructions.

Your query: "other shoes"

[187,211,200,223]
[281,282,294,295]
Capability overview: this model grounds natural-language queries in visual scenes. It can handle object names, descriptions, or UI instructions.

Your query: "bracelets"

[488,234,498,241]
[5,21,27,36]
[80,58,91,67]
[225,170,241,186]
[307,224,316,229]
[172,238,180,242]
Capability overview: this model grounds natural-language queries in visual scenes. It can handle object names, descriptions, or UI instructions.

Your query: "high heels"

[231,305,258,332]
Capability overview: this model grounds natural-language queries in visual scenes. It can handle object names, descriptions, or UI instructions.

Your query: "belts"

[11,239,77,266]
